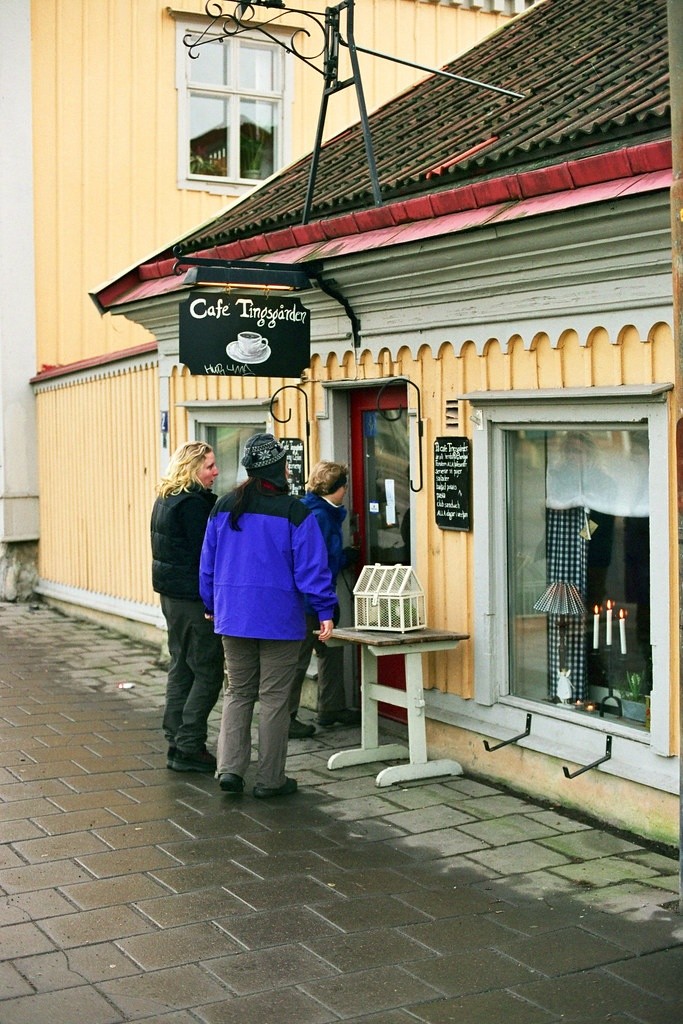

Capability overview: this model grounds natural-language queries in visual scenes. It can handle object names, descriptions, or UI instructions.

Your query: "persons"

[288,462,361,740]
[151,441,218,771]
[199,434,339,799]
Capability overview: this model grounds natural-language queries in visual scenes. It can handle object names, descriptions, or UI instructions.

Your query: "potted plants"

[618,669,651,723]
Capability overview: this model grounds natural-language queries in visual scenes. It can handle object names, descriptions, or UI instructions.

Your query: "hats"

[241,433,286,476]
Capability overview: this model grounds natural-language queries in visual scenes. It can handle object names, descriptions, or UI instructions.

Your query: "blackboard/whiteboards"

[433,436,470,532]
[278,438,305,498]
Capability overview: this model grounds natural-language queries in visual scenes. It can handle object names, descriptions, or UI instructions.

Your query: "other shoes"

[166,746,217,772]
[219,774,245,792]
[253,777,297,799]
[288,719,316,737]
[317,709,361,725]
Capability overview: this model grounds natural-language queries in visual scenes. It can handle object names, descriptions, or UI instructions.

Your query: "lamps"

[533,583,587,710]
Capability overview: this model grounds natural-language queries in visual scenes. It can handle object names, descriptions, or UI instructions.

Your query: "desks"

[313,627,470,788]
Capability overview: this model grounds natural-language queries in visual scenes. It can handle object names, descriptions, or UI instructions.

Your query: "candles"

[592,604,600,648]
[606,599,613,646]
[619,609,627,654]
